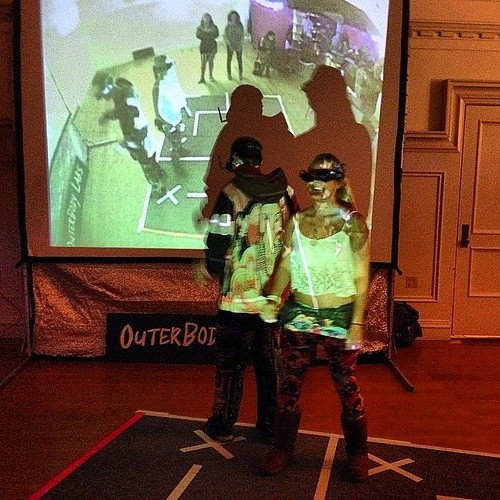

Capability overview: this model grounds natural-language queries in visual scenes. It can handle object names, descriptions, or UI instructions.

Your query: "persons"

[196,12,219,84]
[285,22,383,118]
[257,31,276,77]
[205,135,300,442]
[259,153,371,482]
[93,72,170,200]
[153,55,192,177]
[223,11,245,81]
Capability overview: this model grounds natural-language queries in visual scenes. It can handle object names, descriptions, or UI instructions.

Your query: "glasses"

[298,168,344,182]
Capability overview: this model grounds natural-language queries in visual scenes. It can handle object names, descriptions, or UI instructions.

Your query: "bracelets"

[351,322,364,326]
[265,294,282,304]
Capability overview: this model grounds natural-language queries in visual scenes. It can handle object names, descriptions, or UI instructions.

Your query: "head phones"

[226,143,263,172]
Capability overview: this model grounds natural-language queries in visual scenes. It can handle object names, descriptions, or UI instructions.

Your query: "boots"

[261,407,302,475]
[341,411,368,483]
[205,365,245,439]
[246,376,278,442]
